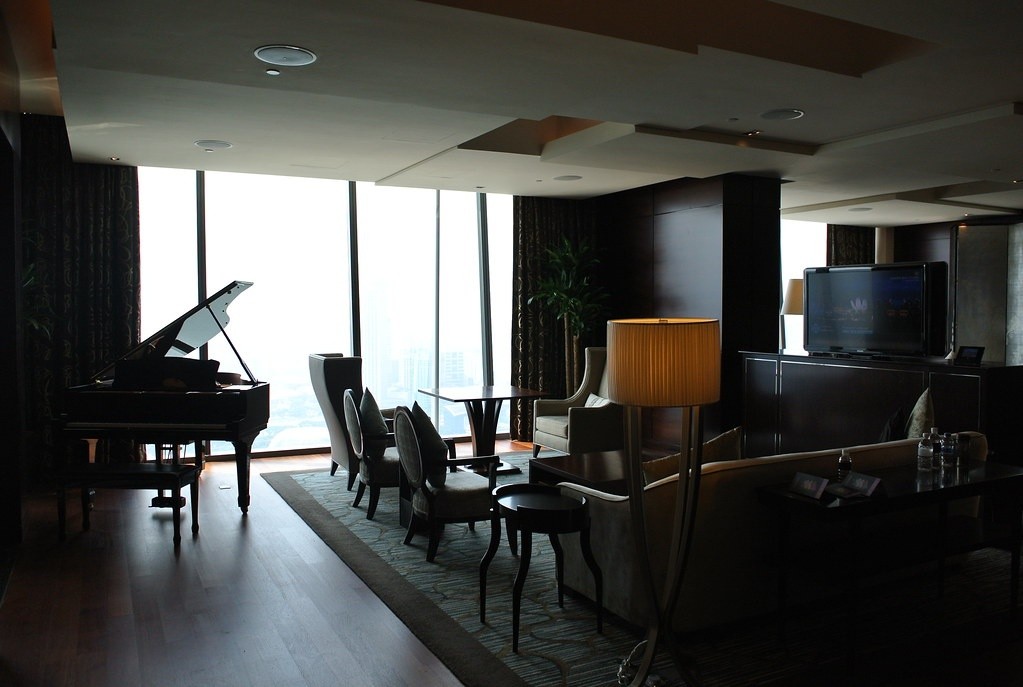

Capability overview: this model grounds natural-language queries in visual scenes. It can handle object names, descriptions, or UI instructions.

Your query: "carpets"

[260,452,1023,687]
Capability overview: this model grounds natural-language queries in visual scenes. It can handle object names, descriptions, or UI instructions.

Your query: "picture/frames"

[954,346,985,368]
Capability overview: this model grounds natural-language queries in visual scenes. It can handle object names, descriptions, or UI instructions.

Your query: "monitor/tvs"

[803,261,929,363]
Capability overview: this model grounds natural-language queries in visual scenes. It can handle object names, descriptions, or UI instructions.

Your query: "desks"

[417,385,553,477]
[479,483,604,654]
[738,346,1023,465]
[755,451,1023,676]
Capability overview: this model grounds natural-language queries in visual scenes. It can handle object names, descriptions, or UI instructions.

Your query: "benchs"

[55,462,199,544]
[555,433,990,637]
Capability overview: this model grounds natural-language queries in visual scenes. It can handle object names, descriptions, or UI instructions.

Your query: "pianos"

[46,280,271,545]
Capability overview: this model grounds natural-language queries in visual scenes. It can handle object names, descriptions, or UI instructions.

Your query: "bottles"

[918,432,933,472]
[928,428,942,470]
[838,449,852,481]
[941,433,959,467]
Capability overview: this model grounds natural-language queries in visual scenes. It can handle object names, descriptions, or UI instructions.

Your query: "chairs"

[308,352,519,564]
[533,347,624,456]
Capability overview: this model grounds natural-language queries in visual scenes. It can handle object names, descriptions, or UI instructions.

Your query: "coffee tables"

[529,447,671,492]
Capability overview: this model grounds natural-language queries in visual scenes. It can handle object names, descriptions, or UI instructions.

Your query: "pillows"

[902,386,935,439]
[644,425,741,486]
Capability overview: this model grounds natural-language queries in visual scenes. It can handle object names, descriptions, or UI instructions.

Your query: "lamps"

[606,315,722,687]
